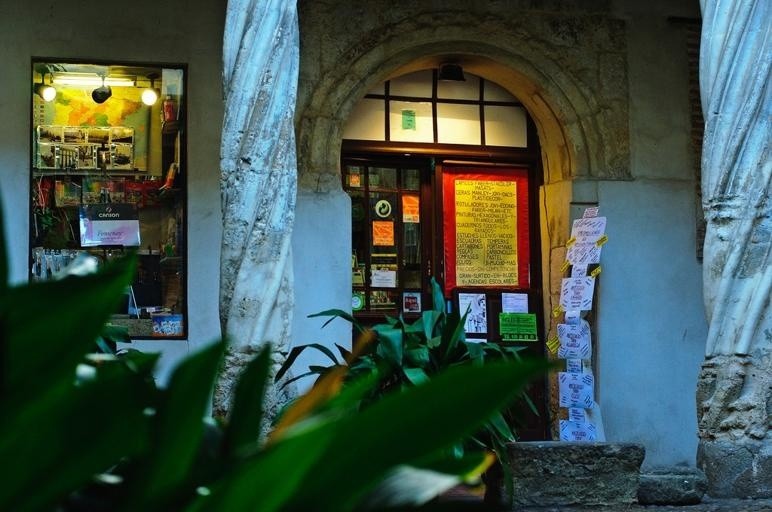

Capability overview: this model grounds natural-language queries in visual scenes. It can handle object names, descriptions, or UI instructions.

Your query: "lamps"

[35,65,161,106]
[438,61,466,81]
